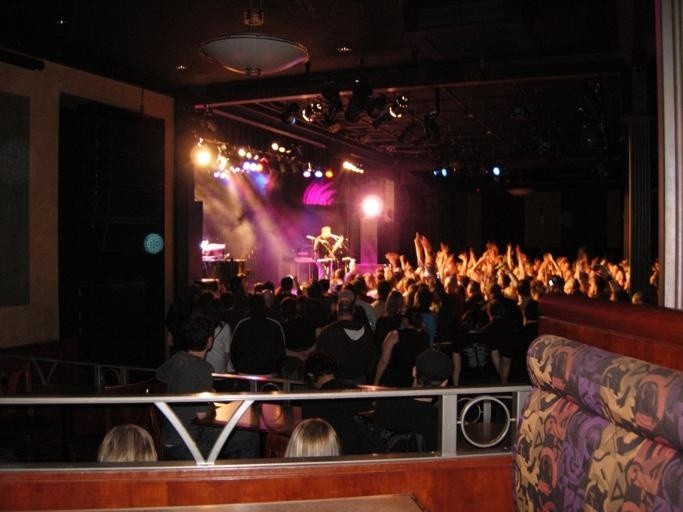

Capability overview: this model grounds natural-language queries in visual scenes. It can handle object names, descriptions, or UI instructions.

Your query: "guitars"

[322,234,344,265]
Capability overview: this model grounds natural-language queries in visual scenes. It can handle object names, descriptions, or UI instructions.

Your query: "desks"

[194,402,302,458]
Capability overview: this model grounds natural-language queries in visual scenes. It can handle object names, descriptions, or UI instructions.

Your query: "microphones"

[305,234,316,240]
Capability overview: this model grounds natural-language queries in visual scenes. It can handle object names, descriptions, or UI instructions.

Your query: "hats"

[151,351,211,413]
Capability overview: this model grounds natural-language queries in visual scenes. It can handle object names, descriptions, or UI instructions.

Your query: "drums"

[317,259,337,279]
[293,245,313,263]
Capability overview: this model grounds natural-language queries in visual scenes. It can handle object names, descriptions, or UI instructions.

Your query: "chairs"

[0,361,167,461]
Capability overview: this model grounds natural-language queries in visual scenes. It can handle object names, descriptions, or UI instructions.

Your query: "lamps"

[389,92,409,120]
[301,94,323,123]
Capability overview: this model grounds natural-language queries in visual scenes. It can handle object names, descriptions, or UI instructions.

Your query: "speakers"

[358,215,383,264]
